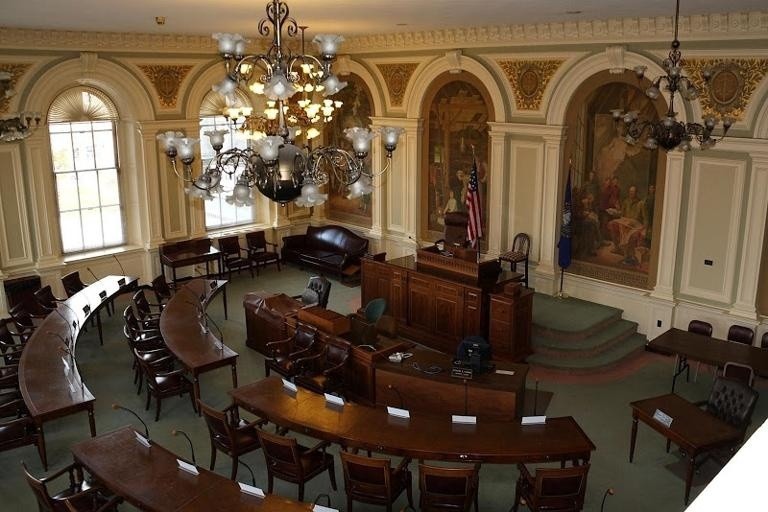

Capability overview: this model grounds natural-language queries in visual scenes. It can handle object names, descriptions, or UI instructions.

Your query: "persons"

[576,172,655,249]
[442,169,471,214]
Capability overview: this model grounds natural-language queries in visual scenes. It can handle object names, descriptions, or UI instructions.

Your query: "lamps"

[156,1,409,210]
[608,0,737,153]
[1,70,47,144]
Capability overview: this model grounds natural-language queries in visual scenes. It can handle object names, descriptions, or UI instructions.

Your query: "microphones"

[308,494,330,510]
[389,384,403,409]
[46,331,71,353]
[315,368,349,404]
[185,266,223,348]
[277,349,297,387]
[601,488,615,512]
[112,403,149,438]
[55,308,72,331]
[228,452,256,487]
[409,236,421,248]
[59,346,84,390]
[533,378,540,416]
[463,379,468,416]
[87,268,98,280]
[172,430,195,464]
[113,254,125,277]
[51,301,78,321]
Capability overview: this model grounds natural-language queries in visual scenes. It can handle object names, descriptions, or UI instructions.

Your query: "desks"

[371,349,533,418]
[160,276,241,416]
[227,375,597,473]
[19,271,142,471]
[67,423,336,512]
[628,394,737,506]
[159,239,222,293]
[645,328,768,396]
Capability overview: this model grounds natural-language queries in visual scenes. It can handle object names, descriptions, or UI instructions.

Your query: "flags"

[557,165,573,269]
[464,157,484,250]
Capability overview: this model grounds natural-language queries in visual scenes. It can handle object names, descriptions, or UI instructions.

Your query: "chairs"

[694,375,758,444]
[500,231,535,287]
[509,457,592,512]
[16,458,127,512]
[293,275,332,315]
[122,271,193,424]
[338,448,415,511]
[293,337,354,391]
[684,320,766,346]
[1,268,89,466]
[263,323,319,379]
[217,234,252,282]
[415,459,482,511]
[195,398,261,480]
[254,423,336,502]
[353,295,392,333]
[246,229,284,274]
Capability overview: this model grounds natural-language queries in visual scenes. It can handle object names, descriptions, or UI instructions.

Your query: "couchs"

[281,225,370,285]
